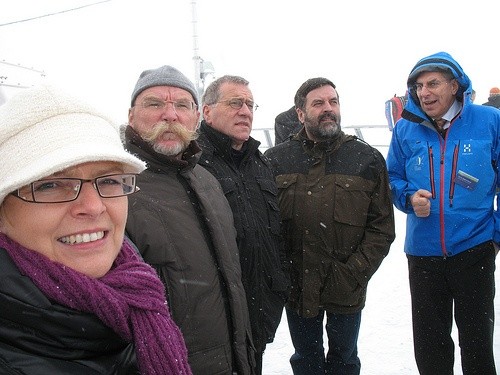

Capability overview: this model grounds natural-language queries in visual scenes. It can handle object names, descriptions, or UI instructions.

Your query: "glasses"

[132,98,197,112]
[8,173,141,204]
[208,98,259,113]
[413,77,456,92]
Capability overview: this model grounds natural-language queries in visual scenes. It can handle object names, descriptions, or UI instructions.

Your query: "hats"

[131,66,199,112]
[0,85,147,207]
[490,88,500,94]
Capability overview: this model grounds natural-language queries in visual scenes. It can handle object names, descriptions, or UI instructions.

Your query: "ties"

[434,119,446,130]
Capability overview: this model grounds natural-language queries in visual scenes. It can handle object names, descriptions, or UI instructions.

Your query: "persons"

[275,106,303,147]
[0,85,192,375]
[191,69,286,375]
[385,52,500,375]
[262,76,396,375]
[122,66,259,375]
